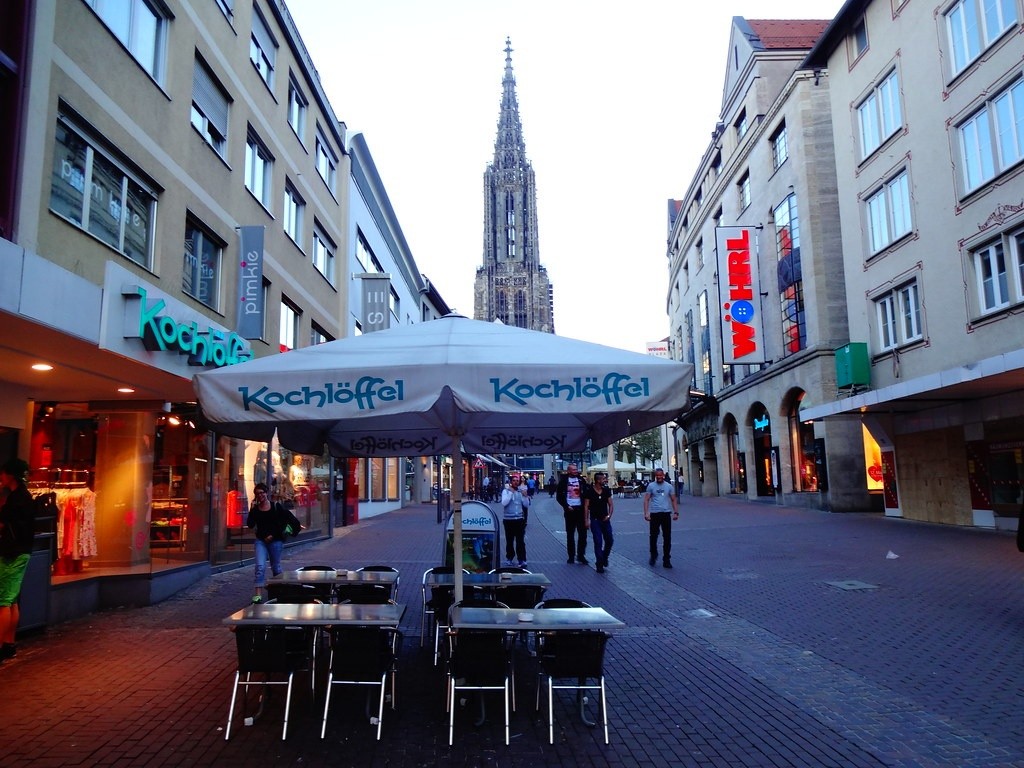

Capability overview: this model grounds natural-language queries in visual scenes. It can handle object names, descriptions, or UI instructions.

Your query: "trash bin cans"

[18,546,53,642]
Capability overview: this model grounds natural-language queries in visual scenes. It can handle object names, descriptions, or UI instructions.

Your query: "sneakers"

[251,595,263,604]
[506,559,514,566]
[518,561,530,569]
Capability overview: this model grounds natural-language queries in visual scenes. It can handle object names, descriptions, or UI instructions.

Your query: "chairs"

[419,565,614,747]
[222,564,402,741]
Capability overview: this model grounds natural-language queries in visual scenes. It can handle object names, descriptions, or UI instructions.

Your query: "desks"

[451,607,627,726]
[230,602,409,726]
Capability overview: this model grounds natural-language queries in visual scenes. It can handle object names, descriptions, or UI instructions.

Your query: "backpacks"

[274,500,302,541]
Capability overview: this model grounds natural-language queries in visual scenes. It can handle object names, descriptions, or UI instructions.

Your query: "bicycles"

[483,486,489,503]
[549,484,555,498]
[487,486,504,503]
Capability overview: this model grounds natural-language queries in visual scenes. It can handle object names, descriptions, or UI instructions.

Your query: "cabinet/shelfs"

[150,463,189,546]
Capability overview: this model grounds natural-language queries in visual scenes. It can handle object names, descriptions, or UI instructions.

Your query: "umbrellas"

[191,312,698,603]
[587,460,654,478]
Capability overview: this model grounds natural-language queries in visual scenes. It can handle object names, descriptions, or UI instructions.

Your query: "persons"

[1,457,36,663]
[643,468,679,569]
[482,473,556,504]
[272,465,286,497]
[501,475,531,568]
[245,482,290,604]
[581,472,615,574]
[289,453,310,530]
[556,465,590,565]
[254,450,268,486]
[618,472,685,499]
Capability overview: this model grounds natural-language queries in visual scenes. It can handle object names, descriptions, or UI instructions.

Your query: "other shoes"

[577,557,589,565]
[567,556,575,565]
[663,559,673,569]
[602,557,608,567]
[595,562,604,573]
[648,556,657,567]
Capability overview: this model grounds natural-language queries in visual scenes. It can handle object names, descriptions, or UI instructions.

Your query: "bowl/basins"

[501,572,512,578]
[336,569,348,575]
[517,612,534,622]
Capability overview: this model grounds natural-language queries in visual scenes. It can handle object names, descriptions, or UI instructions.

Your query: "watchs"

[674,512,679,516]
[607,515,610,519]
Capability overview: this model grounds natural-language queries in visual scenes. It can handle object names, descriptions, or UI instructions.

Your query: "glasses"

[254,491,266,495]
